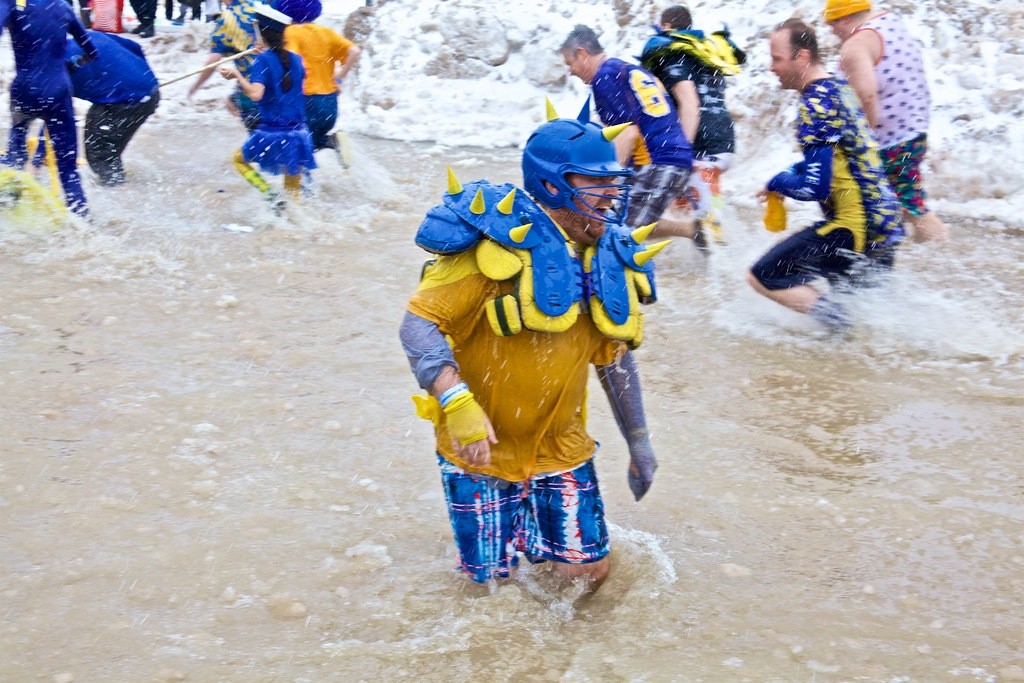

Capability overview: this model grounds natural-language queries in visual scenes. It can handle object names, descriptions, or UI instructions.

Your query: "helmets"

[523,93,635,228]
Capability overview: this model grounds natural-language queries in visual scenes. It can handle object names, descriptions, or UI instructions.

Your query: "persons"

[634,4,747,258]
[29,31,161,186]
[747,18,908,337]
[63,0,258,114]
[397,93,659,592]
[0,0,98,225]
[556,24,713,260]
[283,1,360,166]
[824,0,946,242]
[218,0,319,218]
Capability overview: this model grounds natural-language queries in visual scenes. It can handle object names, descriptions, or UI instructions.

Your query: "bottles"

[763,185,787,233]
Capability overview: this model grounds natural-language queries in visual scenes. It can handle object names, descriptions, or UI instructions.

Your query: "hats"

[824,0,871,25]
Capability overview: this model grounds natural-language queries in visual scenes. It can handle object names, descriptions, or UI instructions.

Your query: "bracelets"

[440,384,468,408]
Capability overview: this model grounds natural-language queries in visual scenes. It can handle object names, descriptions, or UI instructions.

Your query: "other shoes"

[693,218,714,258]
